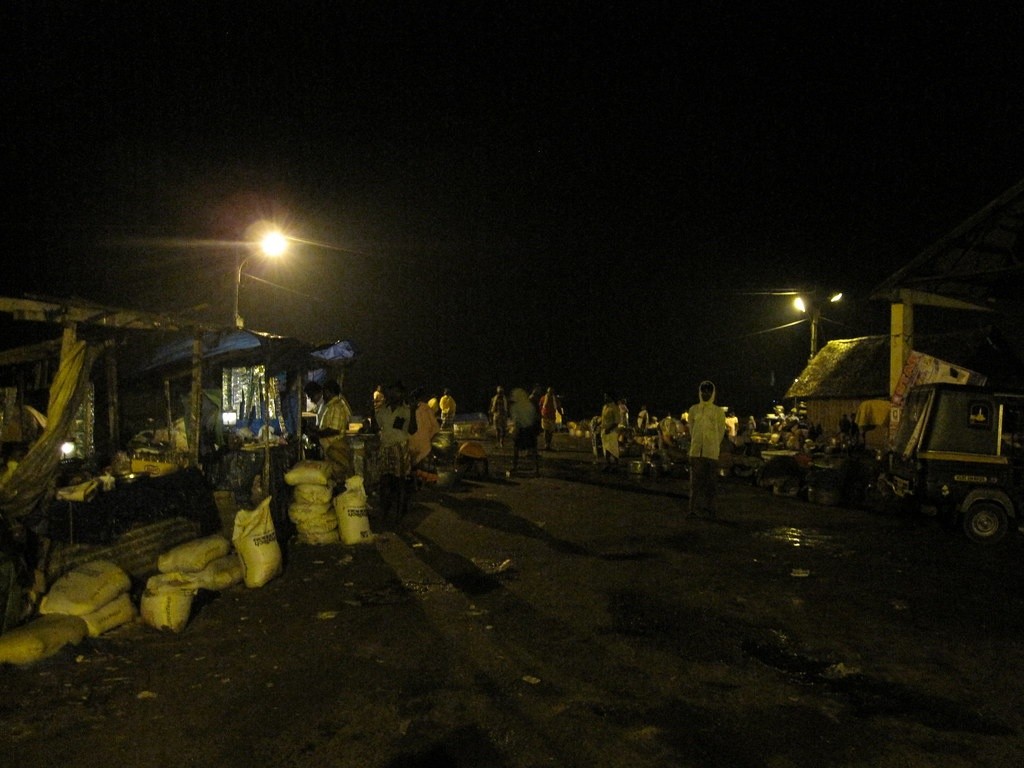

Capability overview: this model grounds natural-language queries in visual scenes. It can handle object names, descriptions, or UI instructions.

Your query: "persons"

[373,383,456,522]
[688,382,726,519]
[488,384,565,478]
[600,392,650,471]
[303,379,354,496]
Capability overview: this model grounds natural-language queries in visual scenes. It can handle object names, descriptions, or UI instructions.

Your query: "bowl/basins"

[629,461,649,474]
[651,462,674,474]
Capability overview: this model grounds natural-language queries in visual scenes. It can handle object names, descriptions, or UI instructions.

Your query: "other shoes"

[611,468,618,473]
[602,467,609,472]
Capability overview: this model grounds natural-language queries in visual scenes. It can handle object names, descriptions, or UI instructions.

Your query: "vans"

[881,382,1024,544]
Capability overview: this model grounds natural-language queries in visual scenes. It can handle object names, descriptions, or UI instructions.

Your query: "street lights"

[790,286,851,360]
[230,230,287,330]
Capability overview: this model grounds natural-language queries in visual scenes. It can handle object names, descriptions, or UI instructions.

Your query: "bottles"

[131,449,184,464]
